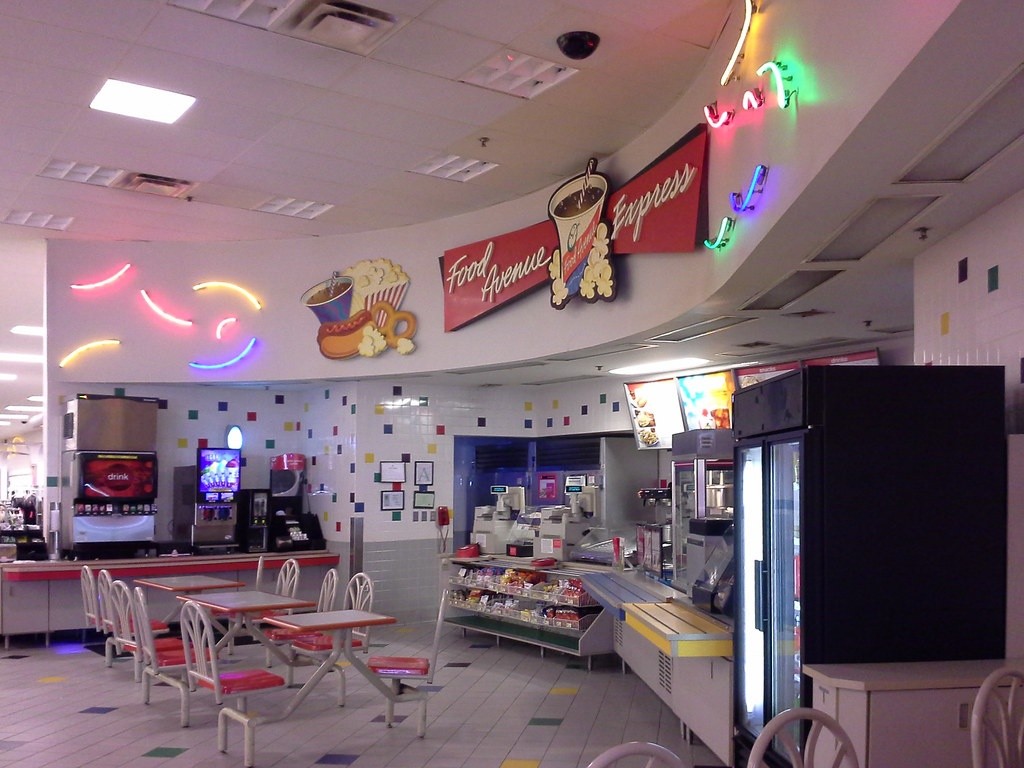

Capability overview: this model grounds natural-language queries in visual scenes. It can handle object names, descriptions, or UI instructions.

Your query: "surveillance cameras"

[556,31,600,59]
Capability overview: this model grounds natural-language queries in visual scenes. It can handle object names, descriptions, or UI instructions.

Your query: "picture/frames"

[414,460,435,486]
[381,490,405,511]
[380,460,406,483]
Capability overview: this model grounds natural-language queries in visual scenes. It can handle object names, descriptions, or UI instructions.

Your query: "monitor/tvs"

[501,487,526,510]
[578,486,596,512]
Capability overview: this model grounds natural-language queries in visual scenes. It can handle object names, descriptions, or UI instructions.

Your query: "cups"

[611,546,624,572]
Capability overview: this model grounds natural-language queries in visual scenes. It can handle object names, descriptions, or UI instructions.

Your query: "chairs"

[587,741,685,768]
[970,667,1024,768]
[747,708,859,768]
[228,558,450,739]
[81,565,287,768]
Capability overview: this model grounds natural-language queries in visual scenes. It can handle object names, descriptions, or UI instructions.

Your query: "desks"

[135,574,246,592]
[263,610,397,633]
[579,572,733,658]
[176,590,316,614]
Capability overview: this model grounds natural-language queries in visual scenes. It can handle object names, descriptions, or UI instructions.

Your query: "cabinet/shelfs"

[0,522,49,561]
[802,661,1024,768]
[443,557,612,670]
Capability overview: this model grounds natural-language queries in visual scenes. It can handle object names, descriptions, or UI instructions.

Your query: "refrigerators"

[730,363,1007,768]
